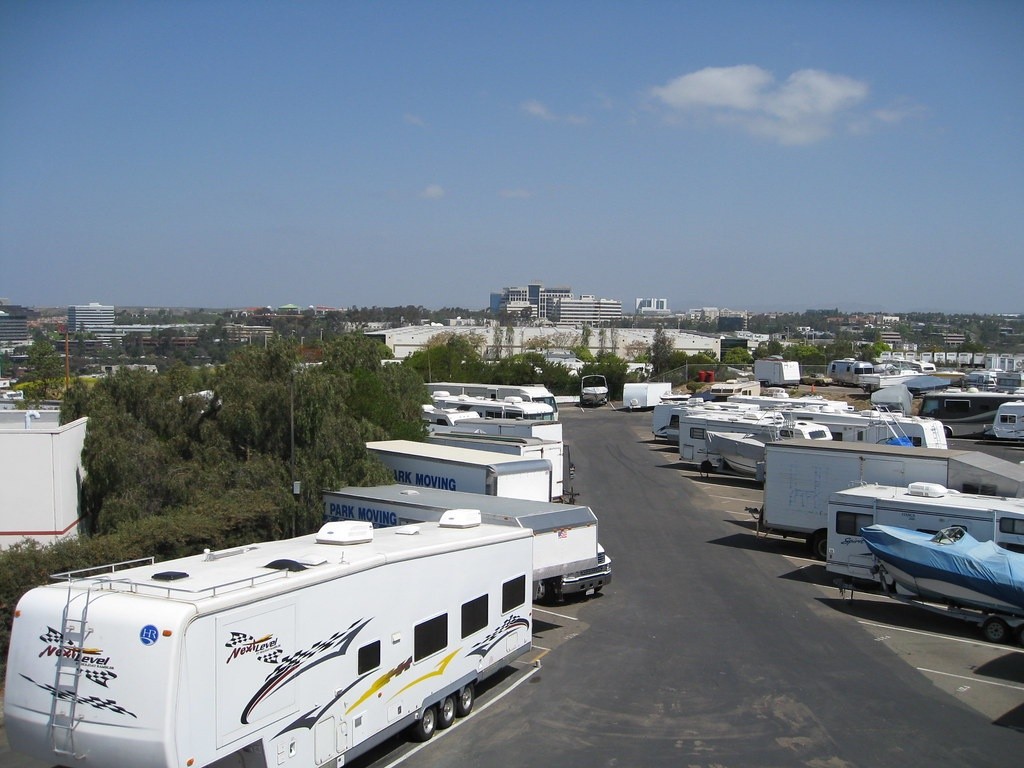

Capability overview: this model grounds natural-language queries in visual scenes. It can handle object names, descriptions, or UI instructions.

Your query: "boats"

[581,374,609,404]
[859,523,1024,616]
[705,430,766,476]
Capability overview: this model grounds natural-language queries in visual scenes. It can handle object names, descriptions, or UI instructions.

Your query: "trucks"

[918,389,1024,439]
[430,390,555,422]
[423,381,559,421]
